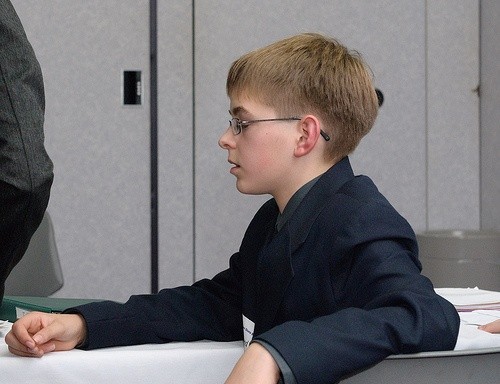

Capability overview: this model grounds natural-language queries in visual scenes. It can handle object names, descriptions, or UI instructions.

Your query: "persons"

[4,32,461,383]
[0,0,54,291]
[478,319,500,333]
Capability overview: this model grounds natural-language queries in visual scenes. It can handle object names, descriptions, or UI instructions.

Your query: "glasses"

[228,117,330,141]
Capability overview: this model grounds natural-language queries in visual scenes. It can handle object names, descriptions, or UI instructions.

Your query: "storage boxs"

[0,295,102,323]
